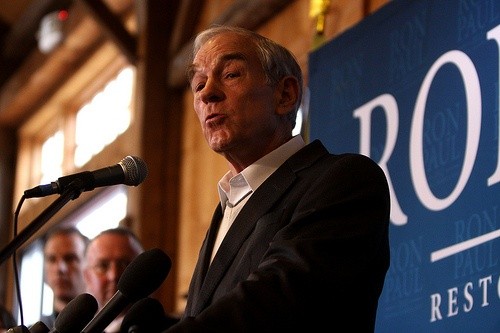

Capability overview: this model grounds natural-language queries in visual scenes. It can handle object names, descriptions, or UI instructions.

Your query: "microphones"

[24,156,147,198]
[3,248,172,333]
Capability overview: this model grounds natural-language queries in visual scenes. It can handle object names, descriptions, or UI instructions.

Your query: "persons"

[178,23,390,333]
[87,228,181,332]
[43,225,90,309]
